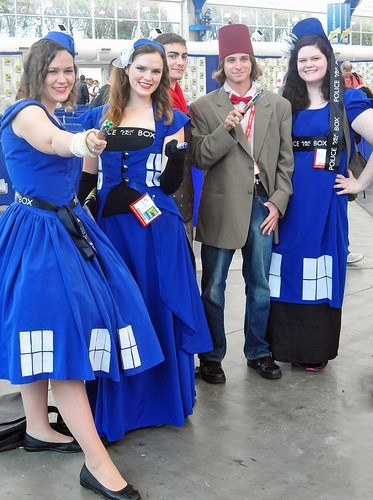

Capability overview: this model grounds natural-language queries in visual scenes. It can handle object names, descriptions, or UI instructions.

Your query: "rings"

[93,148,95,153]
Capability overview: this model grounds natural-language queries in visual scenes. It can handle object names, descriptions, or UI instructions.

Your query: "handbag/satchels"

[347,150,368,202]
[0,392,63,453]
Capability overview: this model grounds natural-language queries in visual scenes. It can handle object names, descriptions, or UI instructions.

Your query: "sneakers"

[346,252,364,263]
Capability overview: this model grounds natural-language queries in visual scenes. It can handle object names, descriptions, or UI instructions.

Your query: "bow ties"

[230,94,252,105]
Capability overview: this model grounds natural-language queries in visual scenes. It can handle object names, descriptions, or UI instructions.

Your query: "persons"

[152,32,190,117]
[0,31,165,500]
[79,74,102,101]
[340,60,365,89]
[347,239,365,263]
[185,24,295,384]
[80,39,213,448]
[268,18,373,372]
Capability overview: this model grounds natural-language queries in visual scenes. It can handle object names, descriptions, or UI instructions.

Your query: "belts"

[254,184,267,197]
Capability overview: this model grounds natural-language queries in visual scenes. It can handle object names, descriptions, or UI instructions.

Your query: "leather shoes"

[247,356,282,380]
[199,360,226,384]
[292,360,328,372]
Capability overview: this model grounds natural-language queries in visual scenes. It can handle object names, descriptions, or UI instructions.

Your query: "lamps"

[148,28,162,40]
[55,24,67,32]
[253,29,263,41]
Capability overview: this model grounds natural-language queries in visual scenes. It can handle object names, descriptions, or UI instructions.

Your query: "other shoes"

[80,463,143,500]
[23,432,84,453]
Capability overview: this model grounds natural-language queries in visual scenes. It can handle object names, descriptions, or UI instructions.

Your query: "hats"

[218,24,254,60]
[291,18,330,45]
